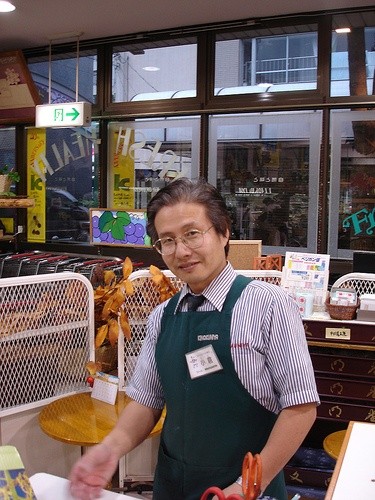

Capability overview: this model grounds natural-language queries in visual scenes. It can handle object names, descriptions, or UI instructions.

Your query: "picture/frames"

[0,48,43,124]
[90,207,153,248]
[229,240,262,269]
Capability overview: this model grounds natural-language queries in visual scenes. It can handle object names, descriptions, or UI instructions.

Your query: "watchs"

[235,476,263,498]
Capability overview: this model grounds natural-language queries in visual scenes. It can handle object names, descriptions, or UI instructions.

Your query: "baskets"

[325,297,360,320]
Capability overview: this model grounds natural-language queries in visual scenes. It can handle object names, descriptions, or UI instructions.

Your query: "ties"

[186,294,208,312]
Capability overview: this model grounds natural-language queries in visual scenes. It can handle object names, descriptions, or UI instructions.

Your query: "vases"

[96,340,118,372]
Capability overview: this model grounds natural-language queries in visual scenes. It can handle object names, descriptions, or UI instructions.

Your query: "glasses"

[153,225,215,256]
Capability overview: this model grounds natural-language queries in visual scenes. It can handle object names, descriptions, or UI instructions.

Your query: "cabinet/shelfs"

[282,311,375,488]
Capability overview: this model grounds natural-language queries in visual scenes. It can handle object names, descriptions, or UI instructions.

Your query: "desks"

[40,392,167,496]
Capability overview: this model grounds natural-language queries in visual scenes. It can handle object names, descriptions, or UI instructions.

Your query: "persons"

[0,218,16,258]
[235,196,255,240]
[254,194,289,247]
[68,176,321,500]
